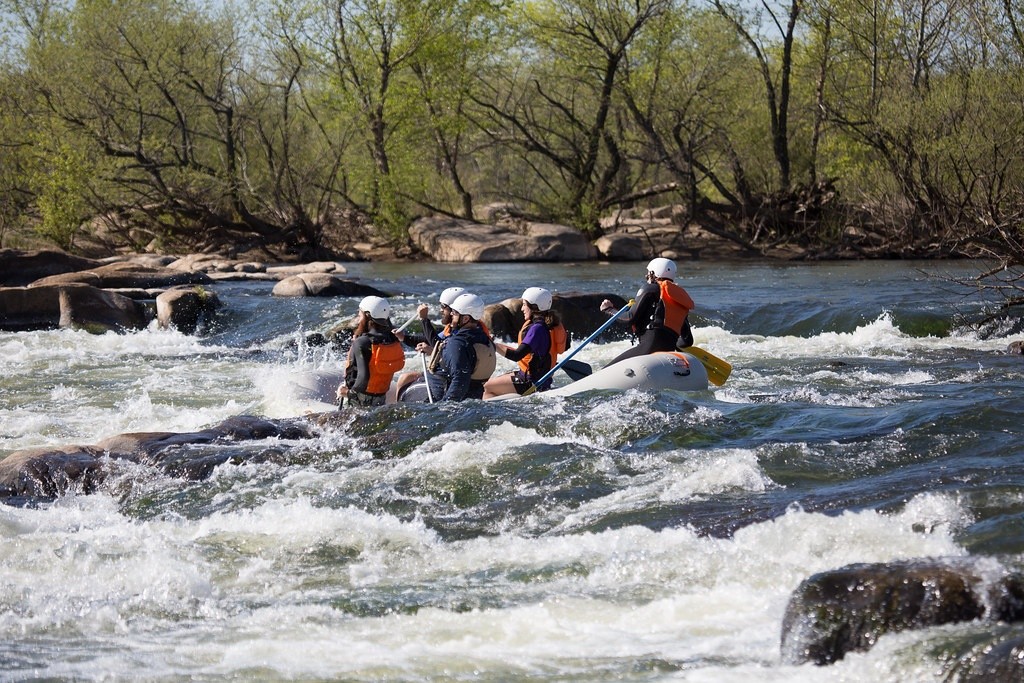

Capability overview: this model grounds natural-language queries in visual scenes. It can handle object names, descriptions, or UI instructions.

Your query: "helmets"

[646,257,677,282]
[360,296,391,320]
[440,287,468,305]
[448,294,485,321]
[521,287,553,311]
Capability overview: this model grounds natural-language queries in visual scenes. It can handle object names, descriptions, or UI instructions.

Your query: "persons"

[479,285,562,401]
[413,293,496,402]
[395,286,477,397]
[599,257,694,374]
[332,294,405,411]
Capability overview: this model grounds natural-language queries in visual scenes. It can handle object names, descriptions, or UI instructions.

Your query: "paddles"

[419,349,436,404]
[519,299,637,397]
[493,341,593,383]
[628,318,732,388]
[337,395,345,412]
[395,313,418,338]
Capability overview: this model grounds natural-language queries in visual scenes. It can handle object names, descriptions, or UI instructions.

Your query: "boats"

[339,346,709,417]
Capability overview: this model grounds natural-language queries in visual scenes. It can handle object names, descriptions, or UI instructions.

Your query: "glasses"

[451,309,459,315]
[440,302,448,309]
